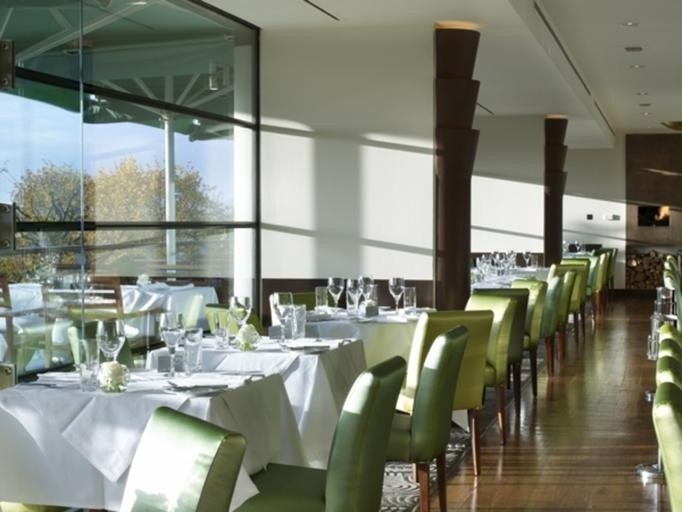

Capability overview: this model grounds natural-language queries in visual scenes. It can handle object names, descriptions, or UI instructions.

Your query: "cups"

[314,286,328,312]
[344,279,361,316]
[183,328,204,374]
[212,310,231,349]
[69,339,100,393]
[403,287,417,316]
[531,255,538,269]
[290,303,308,339]
[368,282,380,305]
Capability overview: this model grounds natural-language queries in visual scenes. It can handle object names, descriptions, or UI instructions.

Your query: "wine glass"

[96,318,127,362]
[574,240,581,254]
[227,295,254,331]
[360,275,373,306]
[273,292,294,344]
[328,277,344,307]
[523,250,532,268]
[159,312,184,381]
[388,278,405,315]
[474,250,519,282]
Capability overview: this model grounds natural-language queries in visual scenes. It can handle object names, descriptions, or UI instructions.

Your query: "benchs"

[42,275,170,363]
[0,273,57,377]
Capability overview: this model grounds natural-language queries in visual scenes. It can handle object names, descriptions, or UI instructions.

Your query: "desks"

[0,369,308,512]
[120,283,221,338]
[268,308,471,434]
[145,333,368,472]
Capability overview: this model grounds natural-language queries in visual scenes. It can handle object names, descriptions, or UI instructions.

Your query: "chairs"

[651,381,682,511]
[540,274,561,378]
[390,308,496,476]
[205,302,264,338]
[608,247,618,313]
[547,262,588,348]
[656,338,682,363]
[386,322,469,512]
[118,405,248,511]
[666,254,681,270]
[662,269,682,320]
[654,356,682,390]
[471,286,531,418]
[235,355,407,512]
[559,258,591,337]
[657,323,682,346]
[7,281,50,338]
[65,318,137,373]
[663,261,681,274]
[601,251,612,316]
[463,292,518,446]
[585,251,607,315]
[510,277,552,397]
[558,268,577,361]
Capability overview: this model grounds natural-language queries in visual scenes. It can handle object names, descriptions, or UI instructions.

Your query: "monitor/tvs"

[638,206,671,226]
